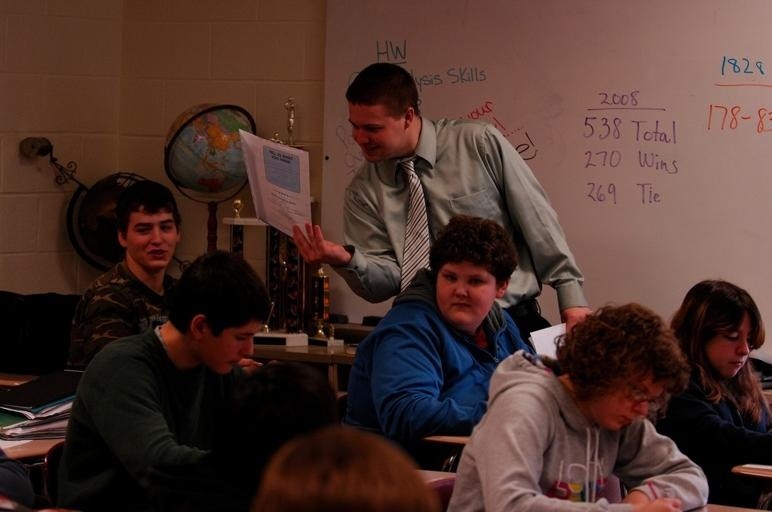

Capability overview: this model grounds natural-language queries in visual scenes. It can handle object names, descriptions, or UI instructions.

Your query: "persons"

[167,359,344,509]
[65,178,279,408]
[1,449,36,505]
[337,216,544,475]
[446,301,710,512]
[249,422,443,512]
[287,59,592,339]
[650,276,771,512]
[50,247,274,510]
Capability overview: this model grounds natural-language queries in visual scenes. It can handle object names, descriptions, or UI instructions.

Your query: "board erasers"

[328,314,348,324]
[362,316,384,327]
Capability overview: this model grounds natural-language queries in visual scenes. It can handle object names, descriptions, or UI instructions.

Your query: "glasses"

[625,377,662,413]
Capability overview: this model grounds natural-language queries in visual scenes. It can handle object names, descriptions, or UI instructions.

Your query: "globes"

[163,103,257,251]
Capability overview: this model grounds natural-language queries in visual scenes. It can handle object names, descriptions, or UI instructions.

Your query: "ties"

[399,159,433,292]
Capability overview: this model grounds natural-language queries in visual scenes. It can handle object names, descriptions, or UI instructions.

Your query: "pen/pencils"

[645,479,661,499]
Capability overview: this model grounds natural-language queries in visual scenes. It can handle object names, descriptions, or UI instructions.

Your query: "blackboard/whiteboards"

[320,2,772,365]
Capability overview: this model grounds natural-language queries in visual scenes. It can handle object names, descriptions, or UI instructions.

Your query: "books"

[0,368,83,443]
[307,336,345,347]
[250,332,310,346]
